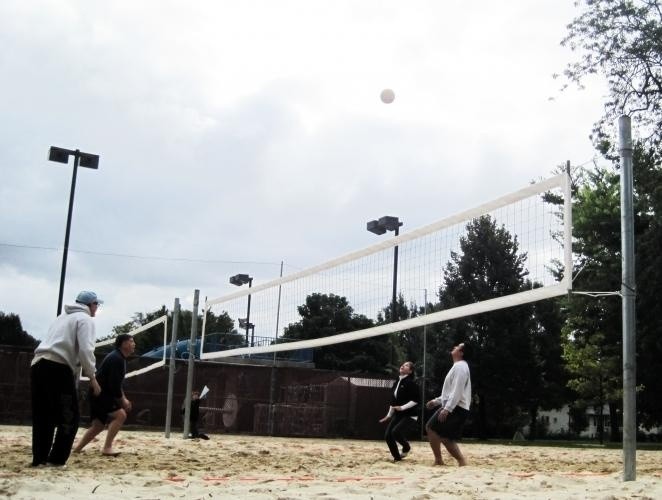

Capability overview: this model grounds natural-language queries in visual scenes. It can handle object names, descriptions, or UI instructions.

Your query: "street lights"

[229,273,252,360]
[47,146,101,317]
[238,321,255,349]
[366,216,403,366]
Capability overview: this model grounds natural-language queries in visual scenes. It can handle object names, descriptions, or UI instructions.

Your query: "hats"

[75,289,106,306]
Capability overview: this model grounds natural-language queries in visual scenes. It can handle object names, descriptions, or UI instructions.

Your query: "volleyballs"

[381,89,394,103]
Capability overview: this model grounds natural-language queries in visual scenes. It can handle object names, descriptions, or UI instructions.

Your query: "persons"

[384,360,420,464]
[424,339,473,468]
[69,335,136,456]
[28,290,104,470]
[188,391,202,439]
